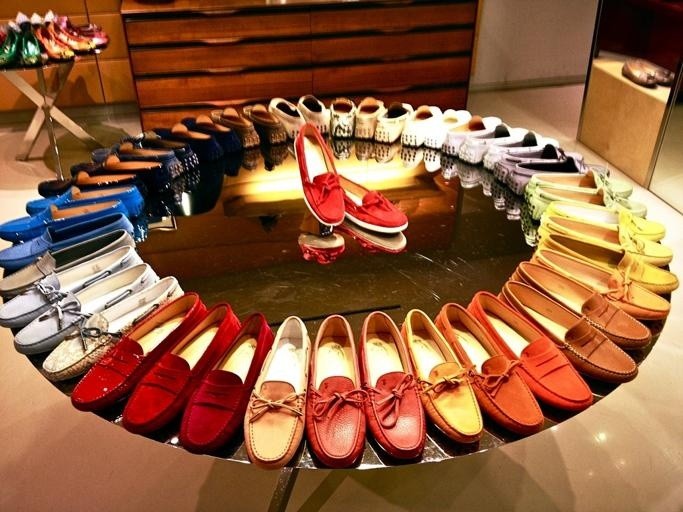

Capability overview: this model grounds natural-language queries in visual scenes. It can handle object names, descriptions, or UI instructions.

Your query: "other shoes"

[2,199,129,240]
[27,146,175,227]
[524,173,674,266]
[337,175,409,234]
[292,122,345,227]
[1,213,135,269]
[124,116,243,179]
[329,97,471,149]
[209,95,329,148]
[459,116,585,177]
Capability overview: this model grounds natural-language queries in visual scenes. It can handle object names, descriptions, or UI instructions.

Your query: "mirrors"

[576,1,683,211]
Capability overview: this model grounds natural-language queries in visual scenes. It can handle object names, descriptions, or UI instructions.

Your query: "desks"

[0,42,106,163]
[1,95,677,512]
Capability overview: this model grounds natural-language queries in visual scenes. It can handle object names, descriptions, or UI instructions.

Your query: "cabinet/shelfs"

[579,58,672,187]
[116,1,484,144]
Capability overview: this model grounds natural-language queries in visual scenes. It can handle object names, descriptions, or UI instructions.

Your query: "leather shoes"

[357,307,428,463]
[11,261,161,356]
[634,55,676,87]
[505,259,654,352]
[242,307,311,470]
[41,274,186,383]
[69,288,208,414]
[431,300,546,437]
[0,8,111,71]
[304,309,366,470]
[620,57,659,89]
[178,308,275,455]
[120,293,241,439]
[493,277,640,388]
[398,304,485,448]
